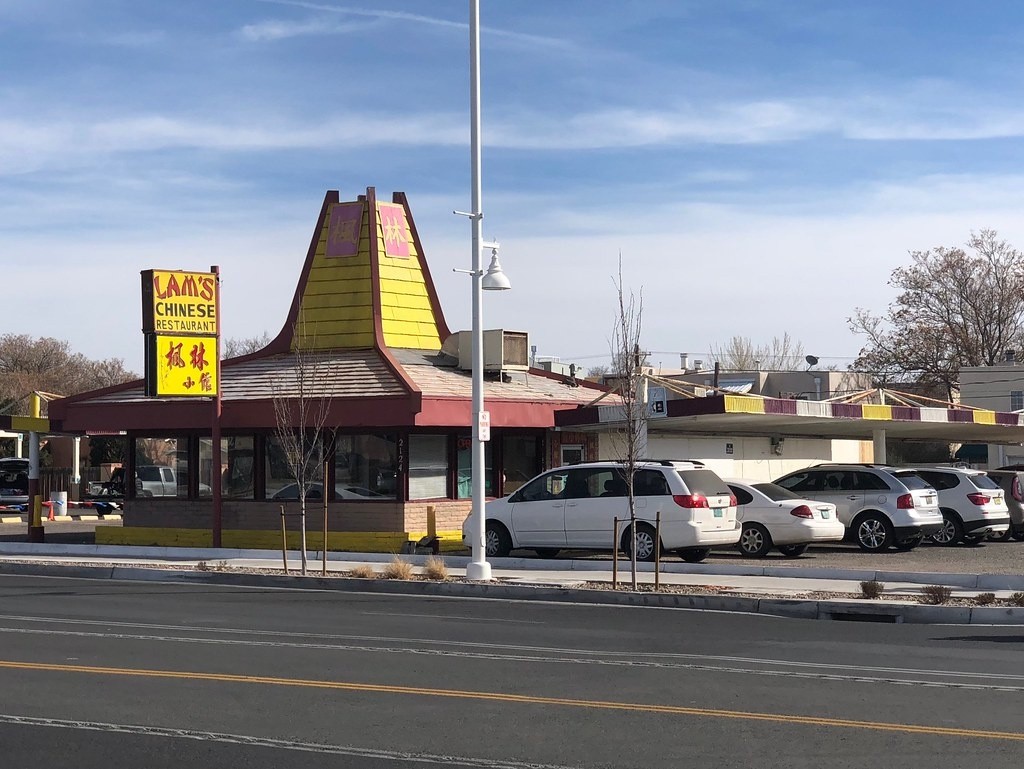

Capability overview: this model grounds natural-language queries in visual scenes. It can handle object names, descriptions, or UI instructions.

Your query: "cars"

[716,478,846,559]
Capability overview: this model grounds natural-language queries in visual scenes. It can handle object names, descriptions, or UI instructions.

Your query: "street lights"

[454,239,511,587]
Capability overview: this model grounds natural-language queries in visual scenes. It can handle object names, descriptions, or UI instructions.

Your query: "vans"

[0,457,30,512]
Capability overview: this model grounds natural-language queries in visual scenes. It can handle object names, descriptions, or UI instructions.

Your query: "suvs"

[459,456,743,560]
[883,466,1011,548]
[978,465,1024,542]
[762,463,945,554]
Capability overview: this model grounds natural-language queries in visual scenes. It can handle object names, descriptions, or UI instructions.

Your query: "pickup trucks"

[88,465,214,516]
